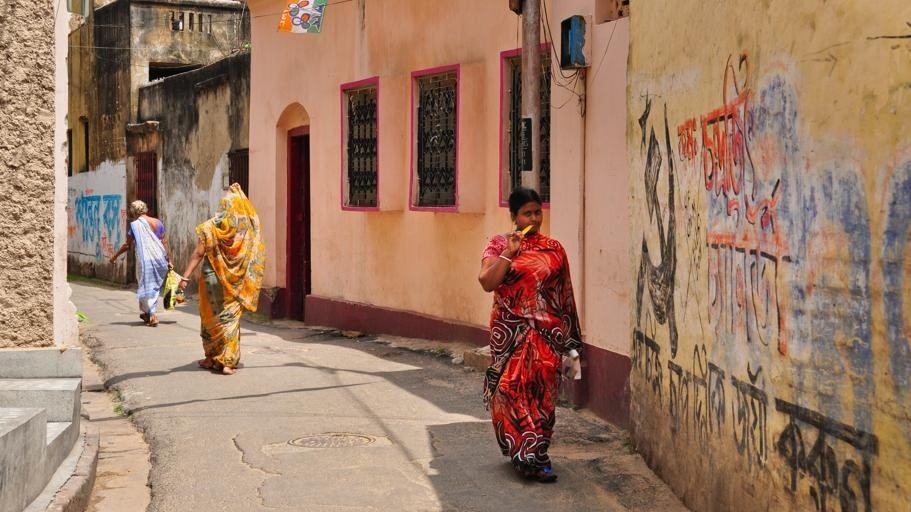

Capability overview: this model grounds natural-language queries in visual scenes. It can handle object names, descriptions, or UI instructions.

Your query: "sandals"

[196,359,220,371]
[224,367,237,375]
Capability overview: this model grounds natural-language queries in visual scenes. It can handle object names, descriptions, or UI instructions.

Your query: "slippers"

[534,466,558,482]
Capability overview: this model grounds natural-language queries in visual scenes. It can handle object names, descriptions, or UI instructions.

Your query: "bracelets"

[181,276,192,282]
[499,254,513,264]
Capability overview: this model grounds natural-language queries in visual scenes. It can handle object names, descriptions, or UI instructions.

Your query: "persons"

[173,180,267,375]
[479,184,588,483]
[110,199,175,327]
